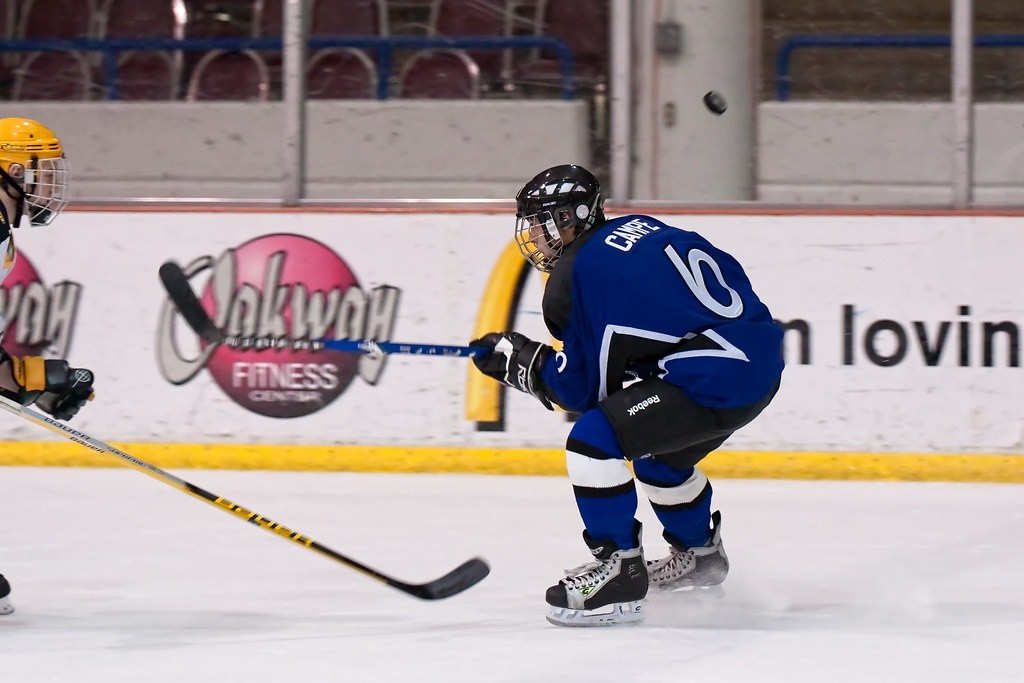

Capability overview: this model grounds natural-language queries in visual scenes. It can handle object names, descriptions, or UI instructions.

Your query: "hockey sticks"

[1,395,492,603]
[157,260,474,359]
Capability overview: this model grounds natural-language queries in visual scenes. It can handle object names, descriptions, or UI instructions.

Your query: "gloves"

[7,354,94,422]
[469,332,555,411]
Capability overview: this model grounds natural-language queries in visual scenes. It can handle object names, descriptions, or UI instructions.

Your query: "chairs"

[0,0,610,139]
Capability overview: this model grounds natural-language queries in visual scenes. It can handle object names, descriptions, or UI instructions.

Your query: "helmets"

[0,117,71,226]
[514,164,602,274]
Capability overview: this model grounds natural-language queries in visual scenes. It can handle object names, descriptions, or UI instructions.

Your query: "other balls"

[703,89,728,115]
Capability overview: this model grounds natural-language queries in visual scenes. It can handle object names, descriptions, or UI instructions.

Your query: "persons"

[0,117,94,616]
[469,163,785,626]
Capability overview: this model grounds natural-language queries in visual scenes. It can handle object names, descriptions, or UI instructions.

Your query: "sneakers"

[544,518,648,626]
[0,574,15,614]
[647,510,730,603]
